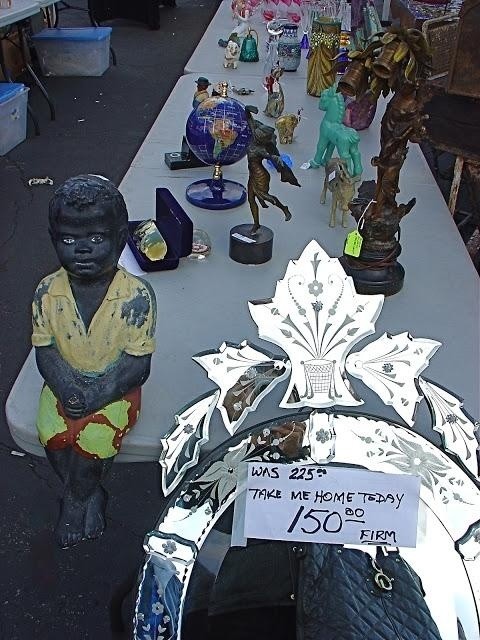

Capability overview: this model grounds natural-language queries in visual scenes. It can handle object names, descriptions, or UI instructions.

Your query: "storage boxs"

[0,83,29,158]
[33,24,112,77]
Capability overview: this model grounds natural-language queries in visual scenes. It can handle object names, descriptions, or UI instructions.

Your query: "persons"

[244,103,303,234]
[30,173,159,552]
[191,76,212,108]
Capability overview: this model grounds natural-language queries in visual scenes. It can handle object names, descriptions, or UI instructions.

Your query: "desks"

[183,2,356,71]
[5,73,480,459]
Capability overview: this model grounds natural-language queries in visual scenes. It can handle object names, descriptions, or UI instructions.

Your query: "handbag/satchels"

[297,541,441,640]
[208,543,300,616]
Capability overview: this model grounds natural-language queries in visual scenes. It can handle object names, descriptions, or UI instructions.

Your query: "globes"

[185,82,255,210]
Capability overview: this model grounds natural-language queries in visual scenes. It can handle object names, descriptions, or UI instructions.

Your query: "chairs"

[418,1,480,228]
